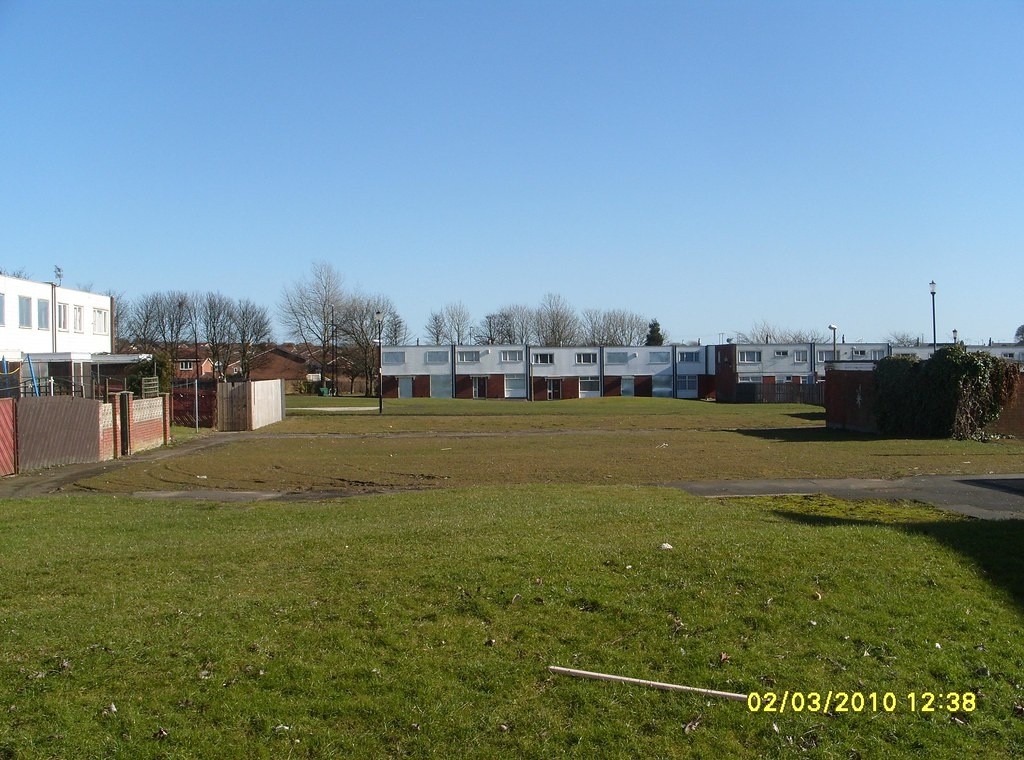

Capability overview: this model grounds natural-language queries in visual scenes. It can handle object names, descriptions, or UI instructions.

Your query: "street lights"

[376,310,383,412]
[828,324,837,360]
[952,329,958,347]
[929,281,936,352]
